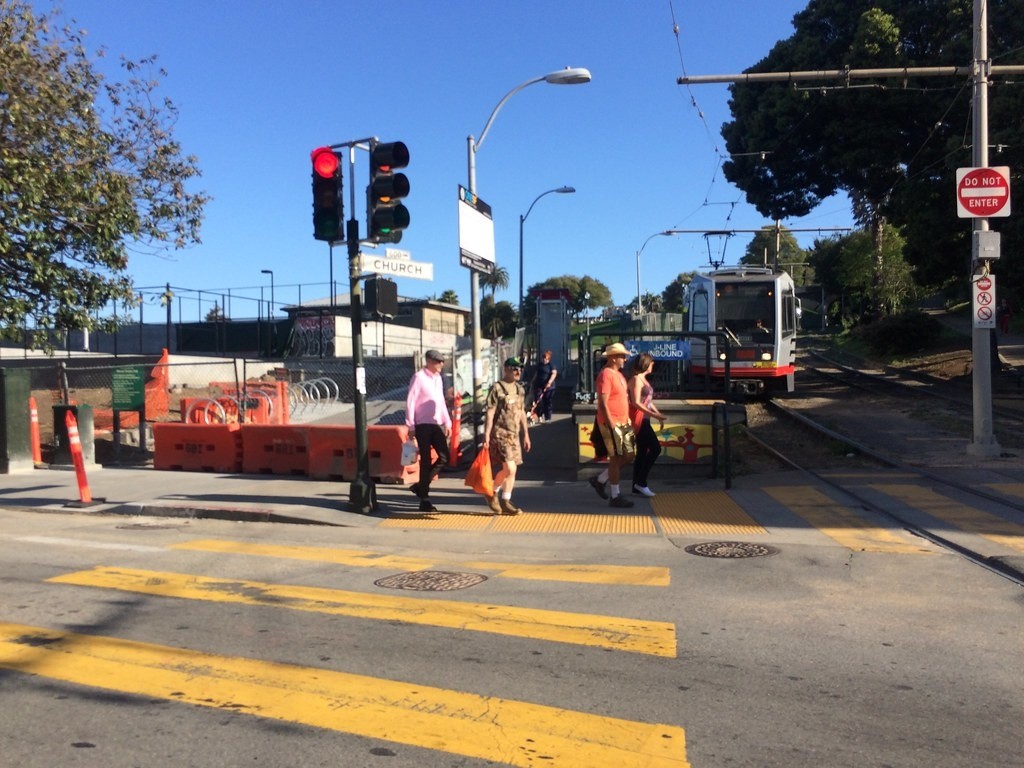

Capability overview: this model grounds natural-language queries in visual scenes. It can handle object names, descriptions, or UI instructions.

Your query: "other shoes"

[634,483,656,497]
[485,492,502,513]
[545,419,551,424]
[498,497,522,515]
[419,500,436,512]
[410,483,423,498]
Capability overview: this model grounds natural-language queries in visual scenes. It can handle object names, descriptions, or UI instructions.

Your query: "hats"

[602,343,631,357]
[426,349,445,363]
[505,356,524,367]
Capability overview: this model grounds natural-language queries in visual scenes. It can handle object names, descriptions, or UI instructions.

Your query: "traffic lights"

[310,146,345,240]
[365,277,397,320]
[366,138,410,244]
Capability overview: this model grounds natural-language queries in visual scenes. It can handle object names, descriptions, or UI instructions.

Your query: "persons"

[406,350,452,512]
[628,354,667,497]
[589,343,634,507]
[755,318,769,333]
[484,357,531,514]
[530,351,557,423]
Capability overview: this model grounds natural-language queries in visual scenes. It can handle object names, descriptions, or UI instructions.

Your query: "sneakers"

[608,495,634,508]
[588,474,607,499]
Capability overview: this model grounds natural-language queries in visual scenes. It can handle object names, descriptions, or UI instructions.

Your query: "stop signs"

[956,166,1011,218]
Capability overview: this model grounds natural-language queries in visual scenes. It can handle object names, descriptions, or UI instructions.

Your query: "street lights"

[636,231,675,315]
[467,65,592,457]
[519,185,577,327]
[261,270,274,355]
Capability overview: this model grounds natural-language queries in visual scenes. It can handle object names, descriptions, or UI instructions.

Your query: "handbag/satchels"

[464,444,494,497]
[628,406,647,437]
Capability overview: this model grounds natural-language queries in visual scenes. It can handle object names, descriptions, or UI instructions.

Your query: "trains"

[682,268,800,395]
